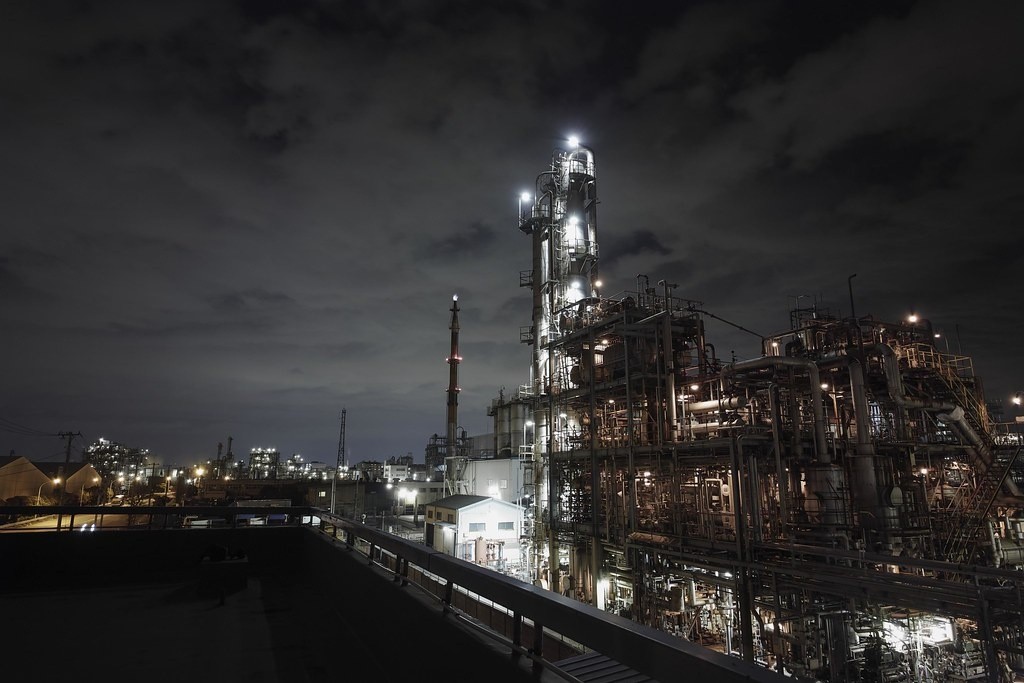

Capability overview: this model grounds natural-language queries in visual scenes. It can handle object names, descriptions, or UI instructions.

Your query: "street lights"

[79,477,99,507]
[127,476,142,496]
[108,476,125,503]
[35,477,61,519]
